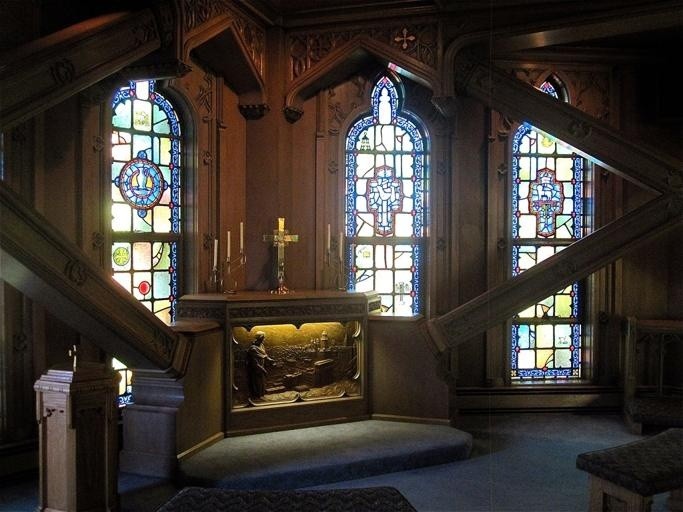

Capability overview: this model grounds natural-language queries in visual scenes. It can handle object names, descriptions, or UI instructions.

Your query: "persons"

[247,331,274,400]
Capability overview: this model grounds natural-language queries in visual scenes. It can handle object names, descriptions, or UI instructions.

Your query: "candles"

[213,220,243,269]
[326,223,343,261]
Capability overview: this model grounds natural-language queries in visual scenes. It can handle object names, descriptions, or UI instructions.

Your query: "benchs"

[153,485,416,511]
[573,425,682,511]
